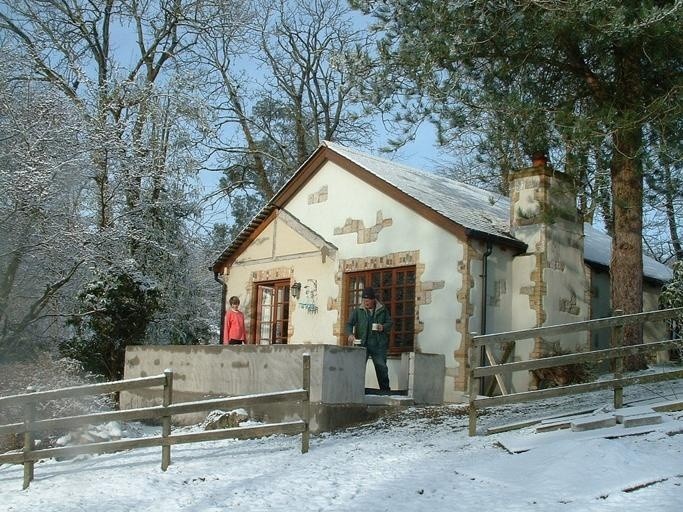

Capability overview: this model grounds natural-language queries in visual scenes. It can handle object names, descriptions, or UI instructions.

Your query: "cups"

[372,323,379,331]
[353,339,362,344]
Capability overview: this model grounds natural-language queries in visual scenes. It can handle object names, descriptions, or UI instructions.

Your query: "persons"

[223,296,248,345]
[345,287,393,395]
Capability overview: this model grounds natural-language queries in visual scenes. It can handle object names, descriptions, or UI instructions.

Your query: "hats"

[362,288,374,299]
